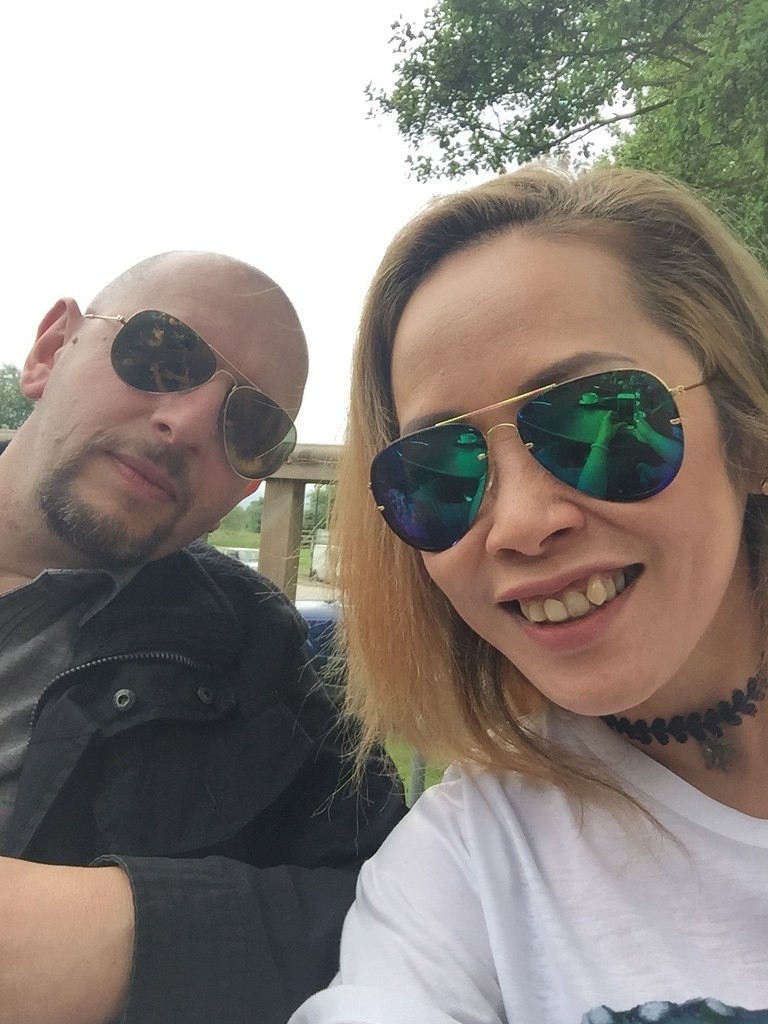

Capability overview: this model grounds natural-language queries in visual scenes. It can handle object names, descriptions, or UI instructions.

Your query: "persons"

[577,409,683,497]
[385,472,485,545]
[151,345,206,393]
[0,250,411,1024]
[288,164,768,1024]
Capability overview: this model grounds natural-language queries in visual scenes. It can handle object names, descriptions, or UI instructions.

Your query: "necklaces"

[600,661,768,771]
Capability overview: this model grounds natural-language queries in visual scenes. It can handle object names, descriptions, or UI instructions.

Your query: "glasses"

[83,308,299,482]
[366,367,719,553]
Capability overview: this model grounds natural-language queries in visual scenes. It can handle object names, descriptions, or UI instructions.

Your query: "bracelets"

[590,444,610,454]
[479,476,485,481]
